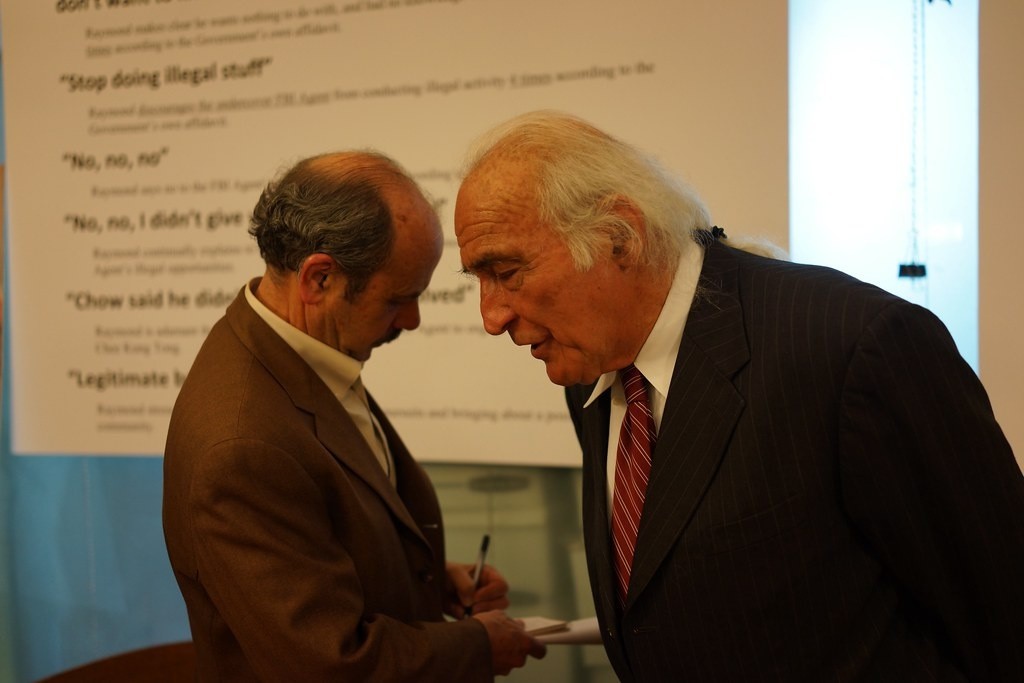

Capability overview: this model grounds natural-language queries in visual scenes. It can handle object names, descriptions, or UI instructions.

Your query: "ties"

[609,365,657,621]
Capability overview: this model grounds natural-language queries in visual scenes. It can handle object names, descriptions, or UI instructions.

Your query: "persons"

[162,150,547,683]
[455,108,1024,683]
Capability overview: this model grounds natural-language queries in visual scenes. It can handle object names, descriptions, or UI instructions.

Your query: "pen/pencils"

[463,533,490,618]
